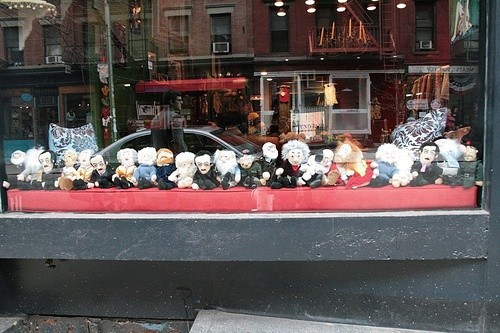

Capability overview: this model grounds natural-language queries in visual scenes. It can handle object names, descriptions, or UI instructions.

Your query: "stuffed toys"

[373,143,396,187]
[175,151,196,188]
[392,149,414,186]
[213,149,240,189]
[114,148,136,190]
[61,148,93,190]
[135,147,156,188]
[57,149,76,189]
[280,139,309,186]
[10,146,44,181]
[262,143,279,186]
[323,133,362,190]
[155,149,176,189]
[238,155,262,189]
[304,149,332,187]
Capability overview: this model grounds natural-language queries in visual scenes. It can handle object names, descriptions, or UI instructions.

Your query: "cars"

[94,126,264,169]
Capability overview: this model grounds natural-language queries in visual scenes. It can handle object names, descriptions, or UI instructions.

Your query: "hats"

[162,90,174,103]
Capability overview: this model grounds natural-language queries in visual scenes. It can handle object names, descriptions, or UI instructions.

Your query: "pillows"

[391,109,447,150]
[48,123,98,169]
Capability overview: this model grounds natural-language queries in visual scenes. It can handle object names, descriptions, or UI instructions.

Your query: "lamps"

[321,82,339,106]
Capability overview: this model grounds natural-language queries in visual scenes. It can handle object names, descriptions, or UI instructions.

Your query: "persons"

[444,140,485,188]
[65,108,75,125]
[73,153,112,189]
[193,154,217,189]
[242,100,269,135]
[152,88,186,150]
[410,145,444,187]
[33,151,57,189]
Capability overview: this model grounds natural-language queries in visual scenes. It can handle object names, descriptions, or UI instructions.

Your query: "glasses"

[175,98,182,101]
